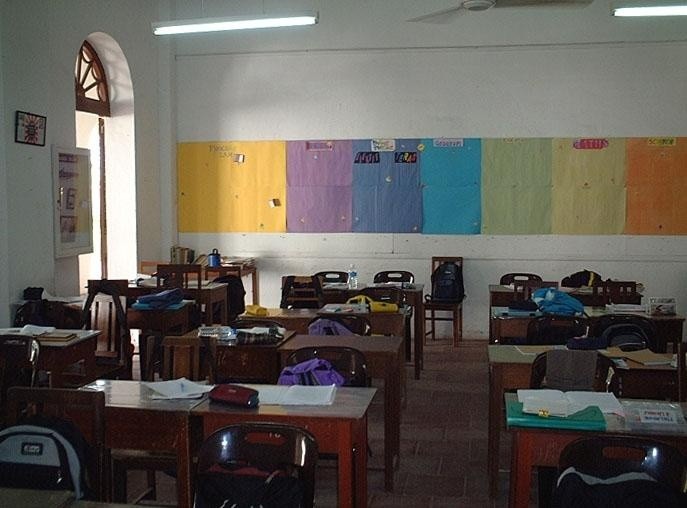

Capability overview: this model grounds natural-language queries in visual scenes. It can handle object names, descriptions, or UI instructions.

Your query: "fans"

[405,1,592,21]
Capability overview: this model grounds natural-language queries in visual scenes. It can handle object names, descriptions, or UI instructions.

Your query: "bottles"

[198,327,236,338]
[348,264,357,290]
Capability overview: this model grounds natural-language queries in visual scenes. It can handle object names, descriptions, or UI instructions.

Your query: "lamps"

[150,10,320,36]
[611,3,687,18]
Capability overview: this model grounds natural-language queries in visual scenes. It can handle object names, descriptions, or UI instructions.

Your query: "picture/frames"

[14,110,47,146]
[51,145,93,259]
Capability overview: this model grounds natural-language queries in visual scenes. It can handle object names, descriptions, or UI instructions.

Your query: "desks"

[489,284,643,307]
[185,266,260,305]
[492,309,686,348]
[182,328,405,493]
[504,392,687,508]
[0,329,102,389]
[488,344,677,497]
[77,380,378,507]
[237,308,406,407]
[325,284,424,380]
[14,297,196,381]
[128,281,228,327]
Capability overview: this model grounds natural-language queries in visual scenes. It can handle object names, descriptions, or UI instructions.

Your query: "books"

[171,247,208,266]
[245,383,337,405]
[517,389,624,417]
[36,333,77,343]
[514,344,567,355]
[625,349,672,365]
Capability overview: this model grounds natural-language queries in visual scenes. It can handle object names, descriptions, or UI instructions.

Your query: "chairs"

[285,346,367,387]
[672,341,687,402]
[281,276,322,308]
[0,335,41,387]
[156,264,202,326]
[226,320,282,329]
[358,287,407,305]
[530,351,621,396]
[315,271,349,283]
[595,312,659,351]
[538,434,687,508]
[47,280,128,389]
[422,257,464,347]
[307,315,371,337]
[514,280,559,305]
[141,261,168,276]
[205,265,242,281]
[500,274,543,285]
[374,271,415,284]
[526,314,593,344]
[192,421,318,508]
[7,387,106,504]
[593,281,636,307]
[109,335,217,507]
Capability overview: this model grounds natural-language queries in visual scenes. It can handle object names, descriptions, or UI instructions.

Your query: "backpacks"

[212,274,246,321]
[532,289,591,320]
[599,322,656,355]
[306,319,355,337]
[425,261,467,304]
[276,358,344,387]
[1,416,91,501]
[552,464,679,507]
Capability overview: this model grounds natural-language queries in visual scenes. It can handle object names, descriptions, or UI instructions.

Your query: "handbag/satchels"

[189,459,314,507]
